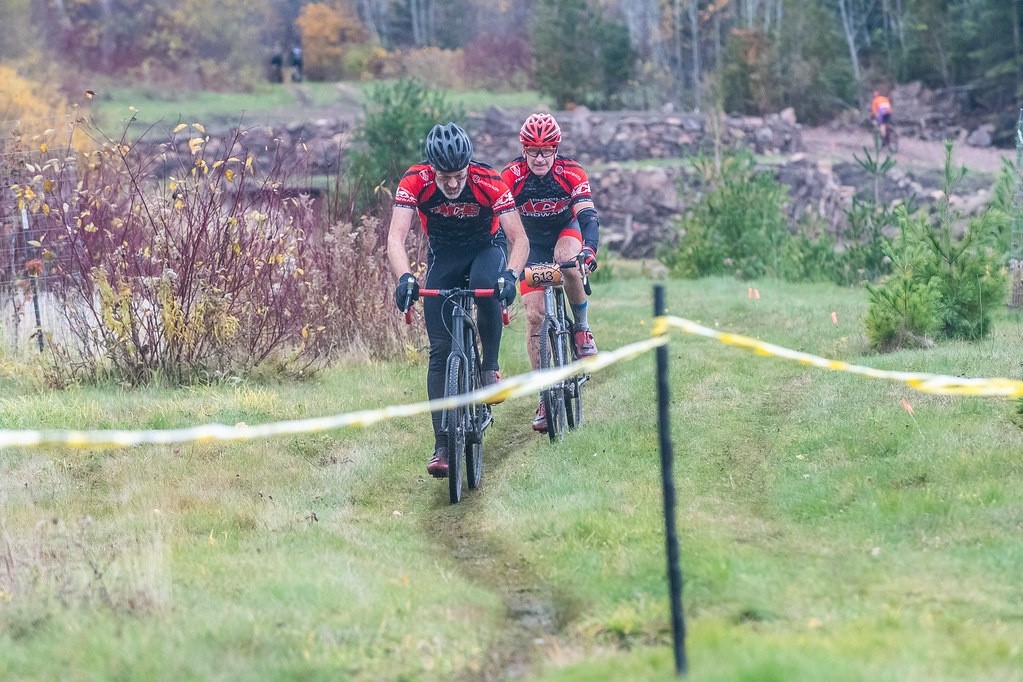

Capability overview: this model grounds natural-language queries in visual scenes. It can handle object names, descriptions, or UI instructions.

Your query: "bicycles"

[871,114,899,155]
[518,250,594,445]
[401,275,511,505]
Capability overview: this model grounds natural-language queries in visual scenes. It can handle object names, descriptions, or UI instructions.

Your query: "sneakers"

[426,447,448,477]
[532,401,547,430]
[480,370,503,405]
[572,322,597,359]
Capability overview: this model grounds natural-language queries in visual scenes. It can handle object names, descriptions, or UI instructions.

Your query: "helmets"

[520,113,561,145]
[425,122,473,172]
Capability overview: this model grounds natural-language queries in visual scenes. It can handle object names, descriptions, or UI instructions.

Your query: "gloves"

[394,272,419,312]
[492,269,519,306]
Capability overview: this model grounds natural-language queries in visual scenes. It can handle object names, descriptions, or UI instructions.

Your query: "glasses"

[524,146,557,157]
[436,167,468,180]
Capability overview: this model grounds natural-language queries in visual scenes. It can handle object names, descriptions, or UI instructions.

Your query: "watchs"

[506,268,520,280]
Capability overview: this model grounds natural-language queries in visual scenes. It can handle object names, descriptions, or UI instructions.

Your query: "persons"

[387,122,530,475]
[264,44,301,84]
[871,90,894,149]
[499,113,600,432]
